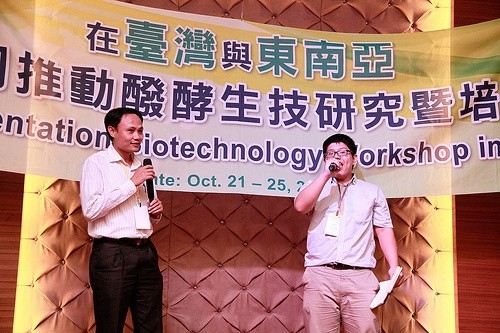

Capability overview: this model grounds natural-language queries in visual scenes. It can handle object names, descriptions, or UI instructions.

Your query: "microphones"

[328,163,341,171]
[143,158,154,203]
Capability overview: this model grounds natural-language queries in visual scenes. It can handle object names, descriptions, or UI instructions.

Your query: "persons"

[293,133,404,333]
[79,106,164,333]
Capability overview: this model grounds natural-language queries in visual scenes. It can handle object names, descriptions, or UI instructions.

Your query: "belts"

[319,262,371,270]
[93,238,151,247]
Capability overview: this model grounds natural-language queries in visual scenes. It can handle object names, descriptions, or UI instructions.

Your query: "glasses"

[325,150,353,158]
